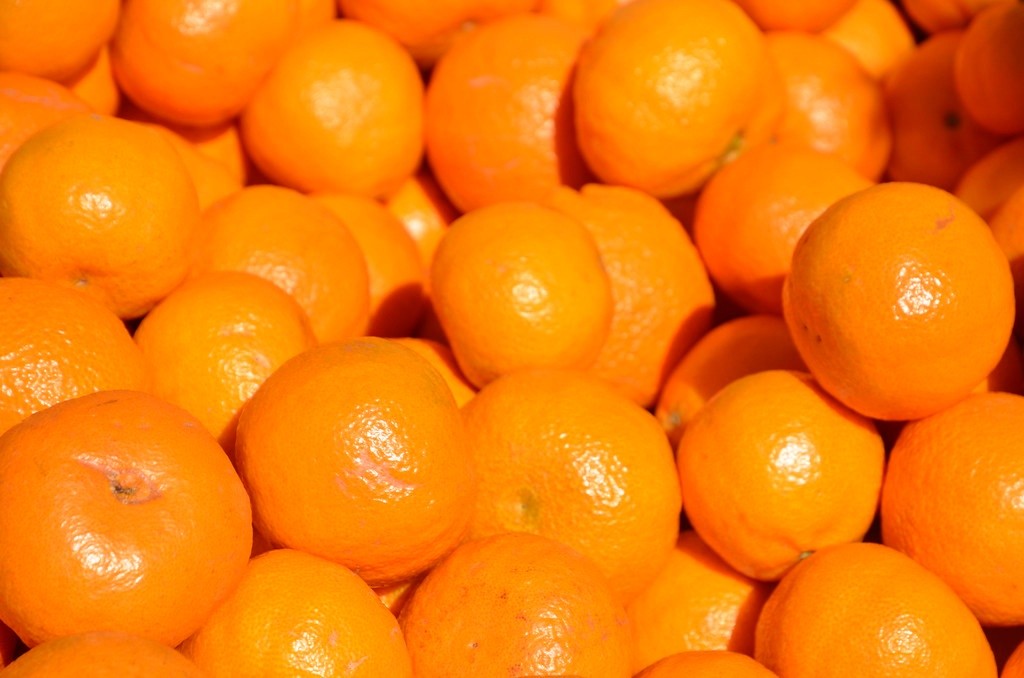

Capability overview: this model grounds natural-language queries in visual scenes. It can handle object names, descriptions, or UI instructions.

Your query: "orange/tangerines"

[0,0,1024,678]
